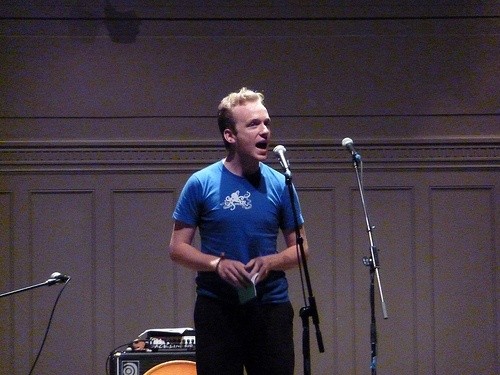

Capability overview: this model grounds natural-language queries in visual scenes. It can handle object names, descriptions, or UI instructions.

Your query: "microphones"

[341,137,361,163]
[49,272,71,281]
[273,144,292,180]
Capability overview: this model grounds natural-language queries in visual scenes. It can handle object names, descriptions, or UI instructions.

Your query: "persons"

[170,87,308,375]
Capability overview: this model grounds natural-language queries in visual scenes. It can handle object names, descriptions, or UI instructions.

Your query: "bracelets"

[216,252,223,273]
